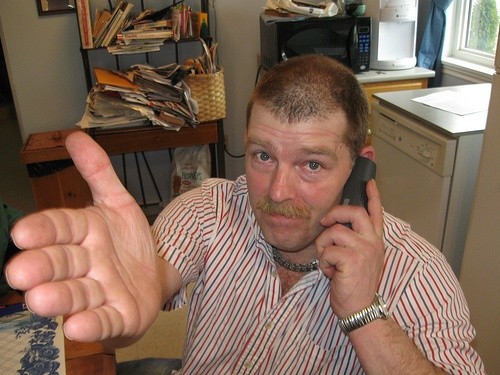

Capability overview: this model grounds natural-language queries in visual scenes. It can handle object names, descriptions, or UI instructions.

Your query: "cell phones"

[341,156,376,231]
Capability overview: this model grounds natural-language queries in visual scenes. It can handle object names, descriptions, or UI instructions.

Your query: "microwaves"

[258,11,371,74]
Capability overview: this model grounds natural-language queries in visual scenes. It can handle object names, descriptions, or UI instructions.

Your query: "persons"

[5,54,485,375]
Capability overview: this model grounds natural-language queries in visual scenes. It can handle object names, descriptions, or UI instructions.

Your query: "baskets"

[184,65,226,123]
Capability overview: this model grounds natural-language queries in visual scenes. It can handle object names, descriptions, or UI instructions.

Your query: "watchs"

[337,293,389,334]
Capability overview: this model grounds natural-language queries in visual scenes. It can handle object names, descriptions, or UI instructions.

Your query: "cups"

[344,0,366,16]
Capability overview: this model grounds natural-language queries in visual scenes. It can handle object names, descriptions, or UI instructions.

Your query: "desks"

[21,121,219,212]
[0,275,116,375]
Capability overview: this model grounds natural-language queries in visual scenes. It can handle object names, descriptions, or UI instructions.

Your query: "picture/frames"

[36,0,77,16]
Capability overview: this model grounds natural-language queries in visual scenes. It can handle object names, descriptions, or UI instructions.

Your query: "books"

[75,0,174,55]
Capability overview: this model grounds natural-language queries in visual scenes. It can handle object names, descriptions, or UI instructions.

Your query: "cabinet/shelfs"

[259,68,429,113]
[76,0,226,208]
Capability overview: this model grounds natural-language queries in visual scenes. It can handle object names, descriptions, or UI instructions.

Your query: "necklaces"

[271,246,319,272]
